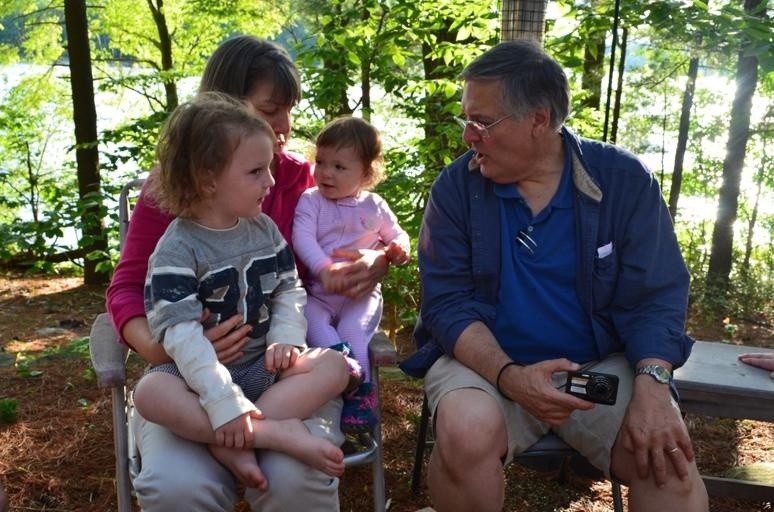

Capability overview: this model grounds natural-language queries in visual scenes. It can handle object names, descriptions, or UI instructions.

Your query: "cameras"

[566,368,619,406]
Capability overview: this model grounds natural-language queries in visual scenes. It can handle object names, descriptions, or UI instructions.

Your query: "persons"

[395,36,709,511]
[292,115,412,471]
[104,34,343,512]
[131,89,353,491]
[737,352,773,379]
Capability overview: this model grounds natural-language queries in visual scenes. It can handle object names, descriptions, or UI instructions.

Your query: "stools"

[671,340,774,508]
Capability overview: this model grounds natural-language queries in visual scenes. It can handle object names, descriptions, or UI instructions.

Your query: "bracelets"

[494,362,526,401]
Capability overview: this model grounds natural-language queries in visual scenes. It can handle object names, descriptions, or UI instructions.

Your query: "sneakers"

[341,382,379,434]
[330,342,364,395]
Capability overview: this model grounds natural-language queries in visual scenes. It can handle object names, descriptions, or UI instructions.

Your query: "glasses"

[454,112,516,138]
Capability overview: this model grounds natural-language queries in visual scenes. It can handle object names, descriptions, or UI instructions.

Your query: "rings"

[667,448,678,454]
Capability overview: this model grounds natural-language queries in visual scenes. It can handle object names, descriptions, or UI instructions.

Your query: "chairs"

[87,177,399,511]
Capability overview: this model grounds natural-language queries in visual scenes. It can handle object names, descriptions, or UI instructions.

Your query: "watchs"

[633,363,672,384]
